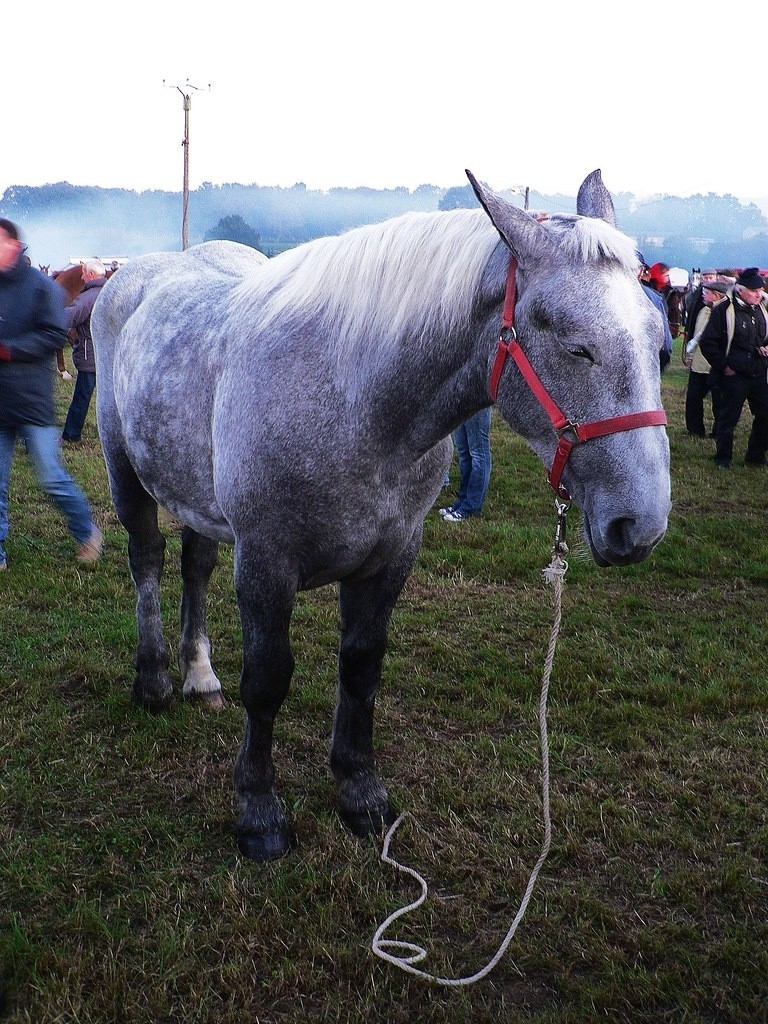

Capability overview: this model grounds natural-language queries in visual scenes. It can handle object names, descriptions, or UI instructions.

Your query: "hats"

[701,268,717,276]
[738,268,766,289]
[701,282,726,293]
[636,251,650,273]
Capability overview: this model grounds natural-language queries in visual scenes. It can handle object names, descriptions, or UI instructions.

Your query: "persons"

[437,406,492,521]
[61,259,120,443]
[633,249,768,470]
[0,218,102,569]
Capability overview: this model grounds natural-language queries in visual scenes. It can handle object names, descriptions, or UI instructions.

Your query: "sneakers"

[438,506,454,515]
[443,510,465,522]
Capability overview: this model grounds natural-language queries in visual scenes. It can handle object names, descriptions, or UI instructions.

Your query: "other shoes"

[75,524,103,563]
[745,456,768,467]
[718,461,733,471]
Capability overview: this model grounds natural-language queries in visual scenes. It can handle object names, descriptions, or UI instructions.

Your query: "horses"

[88,169,672,861]
